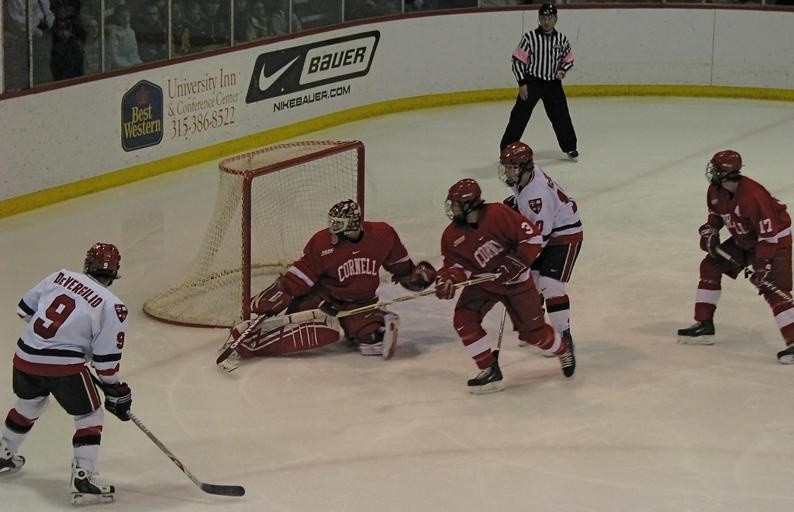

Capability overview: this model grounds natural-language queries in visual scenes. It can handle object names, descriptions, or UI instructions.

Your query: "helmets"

[327,200,362,233]
[498,142,534,184]
[83,243,121,277]
[706,150,741,185]
[444,179,481,222]
[539,3,557,18]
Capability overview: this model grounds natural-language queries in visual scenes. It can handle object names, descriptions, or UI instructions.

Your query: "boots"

[466,360,503,388]
[566,148,579,159]
[69,457,116,496]
[558,342,576,377]
[776,343,794,358]
[360,332,386,349]
[677,319,716,339]
[0,438,25,469]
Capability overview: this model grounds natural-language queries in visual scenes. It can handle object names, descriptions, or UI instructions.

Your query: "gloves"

[495,257,527,283]
[103,383,132,420]
[700,225,721,252]
[248,281,293,316]
[398,261,438,292]
[749,261,776,290]
[433,274,459,301]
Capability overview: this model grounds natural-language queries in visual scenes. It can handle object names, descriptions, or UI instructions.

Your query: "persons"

[678,149,794,359]
[435,177,576,387]
[500,4,578,158]
[223,199,437,360]
[497,142,584,334]
[0,241,132,495]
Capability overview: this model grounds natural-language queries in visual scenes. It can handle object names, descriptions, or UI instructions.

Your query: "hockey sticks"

[318,272,502,318]
[86,363,245,496]
[216,314,267,375]
